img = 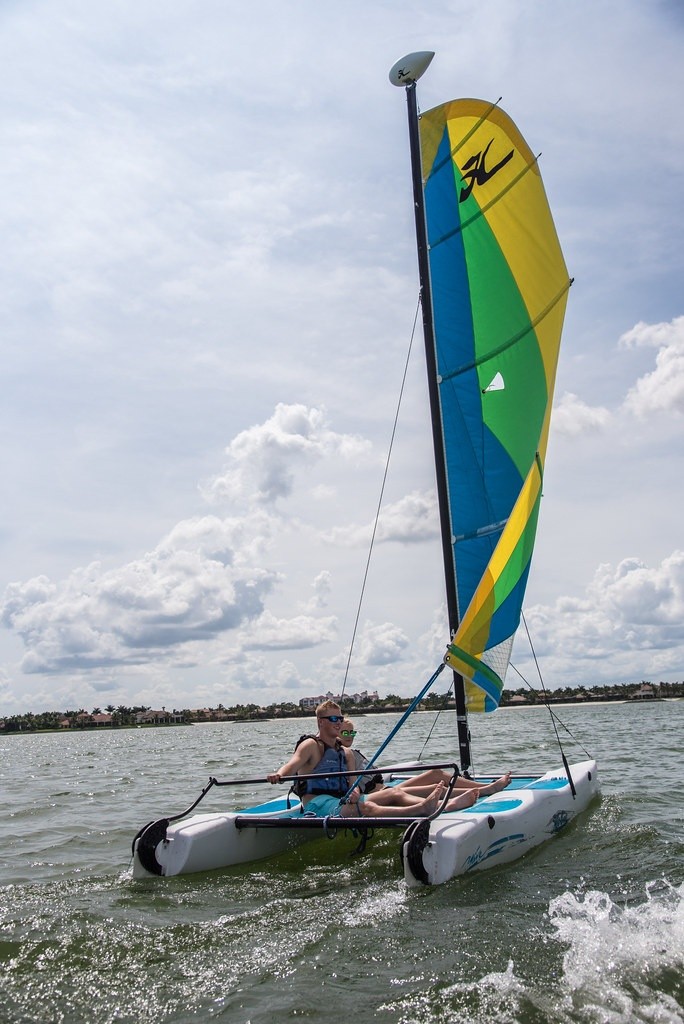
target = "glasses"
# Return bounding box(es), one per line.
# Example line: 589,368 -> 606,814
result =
320,716 -> 344,722
340,730 -> 357,737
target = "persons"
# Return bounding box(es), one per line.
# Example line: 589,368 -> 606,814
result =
335,717 -> 514,800
266,700 -> 479,819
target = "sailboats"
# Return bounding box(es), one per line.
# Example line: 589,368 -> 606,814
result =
128,46 -> 601,898
